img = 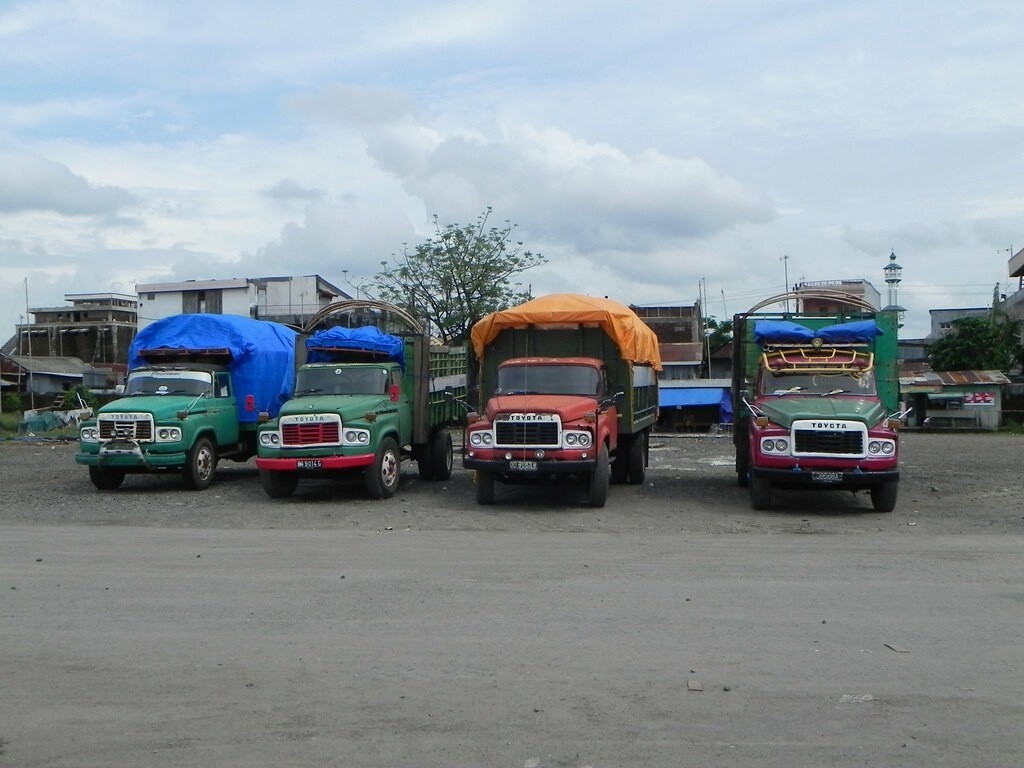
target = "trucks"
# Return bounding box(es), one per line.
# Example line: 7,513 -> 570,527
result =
247,320 -> 467,499
733,313 -> 907,510
75,315 -> 298,489
446,297 -> 658,506
